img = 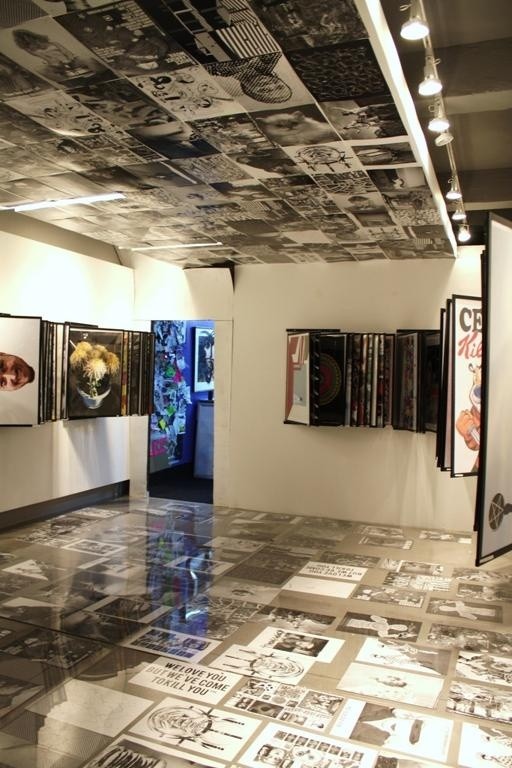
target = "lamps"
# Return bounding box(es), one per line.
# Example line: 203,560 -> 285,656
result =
398,1 -> 471,244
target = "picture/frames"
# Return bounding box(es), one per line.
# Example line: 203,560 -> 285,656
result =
0,312 -> 157,429
285,293 -> 482,479
191,325 -> 214,394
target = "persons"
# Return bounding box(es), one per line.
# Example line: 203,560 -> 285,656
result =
0,352 -> 35,391
62,609 -> 136,644
204,47 -> 292,106
12,29 -> 79,69
345,196 -> 387,216
449,655 -> 512,768
284,637 -> 329,656
236,156 -> 296,174
366,640 -> 452,675
347,615 -> 408,639
439,600 -> 495,620
329,102 -> 402,133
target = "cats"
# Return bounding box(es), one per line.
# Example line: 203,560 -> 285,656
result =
254,110 -> 336,146
